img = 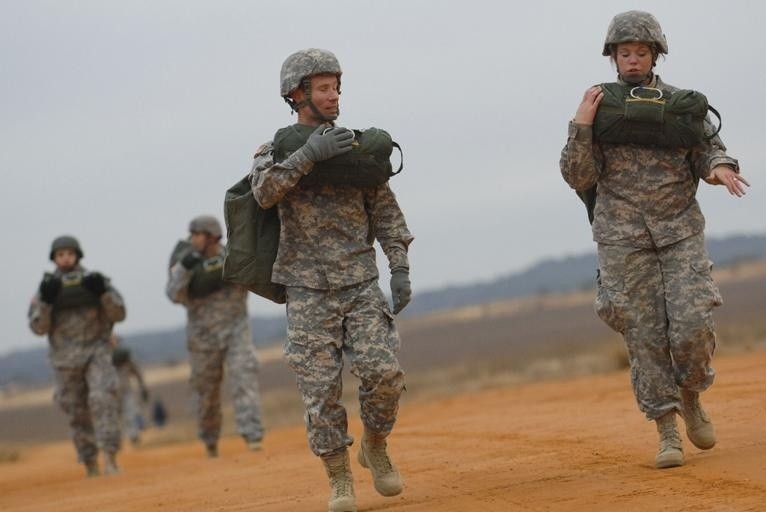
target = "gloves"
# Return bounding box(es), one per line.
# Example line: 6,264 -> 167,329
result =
40,279 -> 61,302
183,252 -> 202,270
82,275 -> 105,295
390,272 -> 411,314
302,124 -> 352,162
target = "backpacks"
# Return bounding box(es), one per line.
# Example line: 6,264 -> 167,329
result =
223,173 -> 286,304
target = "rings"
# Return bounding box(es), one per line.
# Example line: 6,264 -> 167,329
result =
731,177 -> 737,181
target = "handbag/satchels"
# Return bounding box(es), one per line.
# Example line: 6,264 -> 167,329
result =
55,281 -> 92,306
592,83 -> 708,147
188,257 -> 228,297
273,124 -> 392,187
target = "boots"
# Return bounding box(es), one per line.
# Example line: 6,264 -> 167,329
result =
322,450 -> 355,512
678,389 -> 715,449
656,410 -> 684,467
358,425 -> 402,495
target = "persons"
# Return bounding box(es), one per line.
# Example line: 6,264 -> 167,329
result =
163,216 -> 267,459
109,334 -> 149,447
26,237 -> 127,478
249,47 -> 420,511
557,9 -> 750,469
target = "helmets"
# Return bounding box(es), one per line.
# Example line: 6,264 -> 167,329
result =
602,11 -> 668,56
280,49 -> 341,96
50,236 -> 82,259
190,216 -> 222,237
